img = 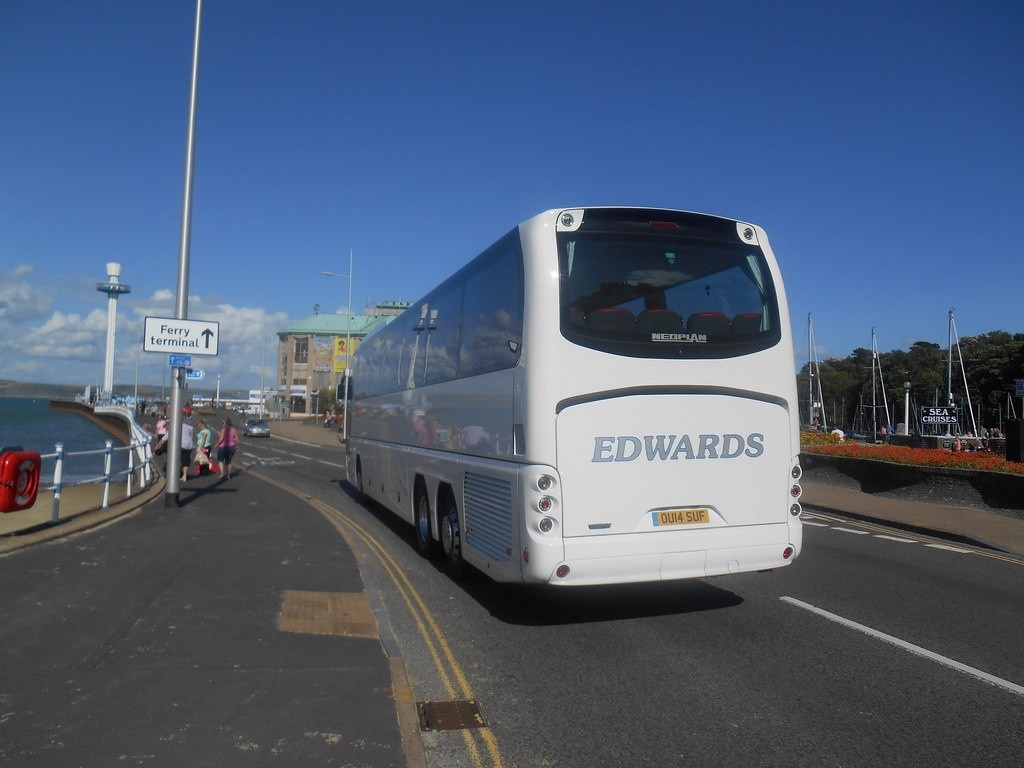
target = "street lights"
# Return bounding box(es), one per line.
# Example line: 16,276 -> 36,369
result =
321,247 -> 349,443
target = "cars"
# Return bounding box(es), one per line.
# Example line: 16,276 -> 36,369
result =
238,417 -> 271,438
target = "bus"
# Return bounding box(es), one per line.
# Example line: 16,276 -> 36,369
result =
336,204 -> 808,591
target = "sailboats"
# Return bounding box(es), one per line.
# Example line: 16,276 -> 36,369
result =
799,305 -> 1006,451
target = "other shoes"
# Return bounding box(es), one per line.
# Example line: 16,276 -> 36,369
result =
180,477 -> 186,481
226,477 -> 230,480
218,477 -> 224,479
200,472 -> 209,475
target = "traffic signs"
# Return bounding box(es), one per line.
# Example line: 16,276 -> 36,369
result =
142,316 -> 220,357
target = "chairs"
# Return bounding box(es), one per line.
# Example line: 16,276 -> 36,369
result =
732,313 -> 762,333
569,306 -> 586,328
587,308 -> 636,333
687,312 -> 730,336
636,308 -> 684,335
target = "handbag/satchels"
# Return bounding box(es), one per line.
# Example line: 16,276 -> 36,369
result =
194,448 -> 209,465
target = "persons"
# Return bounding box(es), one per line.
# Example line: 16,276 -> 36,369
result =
212,397 -> 215,407
140,400 -> 146,416
881,424 -> 892,441
945,425 -> 1003,452
338,340 -> 344,352
196,420 -> 212,477
143,417 -> 193,482
151,405 -> 157,418
183,402 -> 193,419
830,422 -> 847,442
214,418 -> 240,480
90,396 -> 93,405
325,411 -> 332,427
809,417 -> 823,434
